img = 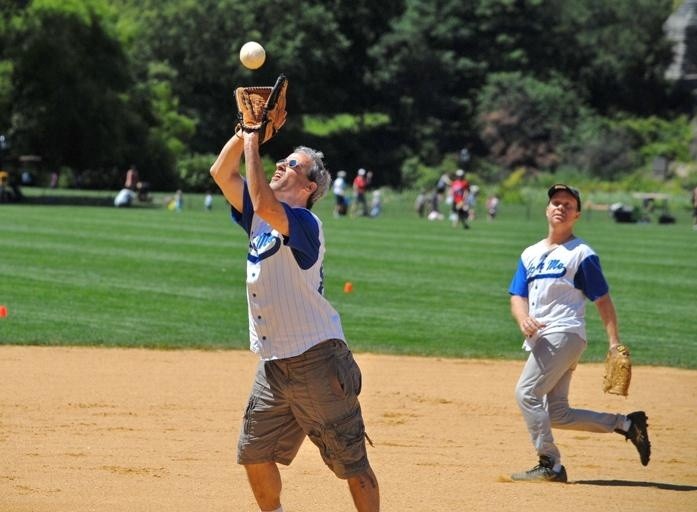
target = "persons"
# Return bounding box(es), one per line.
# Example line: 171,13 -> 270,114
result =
506,183 -> 650,481
331,170 -> 348,218
412,168 -> 503,230
351,168 -> 372,216
369,185 -> 381,216
114,185 -> 138,207
174,182 -> 184,212
124,165 -> 139,192
203,190 -> 212,211
689,186 -> 696,232
211,75 -> 382,512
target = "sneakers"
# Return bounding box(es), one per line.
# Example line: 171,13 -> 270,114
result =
511,454 -> 568,482
615,411 -> 651,466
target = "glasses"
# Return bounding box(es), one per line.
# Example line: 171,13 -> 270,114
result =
278,159 -> 302,171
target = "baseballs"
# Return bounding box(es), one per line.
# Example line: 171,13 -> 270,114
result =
239,42 -> 265,70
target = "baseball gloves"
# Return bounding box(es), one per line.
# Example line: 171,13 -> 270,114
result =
232,73 -> 288,145
602,345 -> 630,395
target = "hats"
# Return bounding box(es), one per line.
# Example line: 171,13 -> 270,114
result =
548,183 -> 581,210
